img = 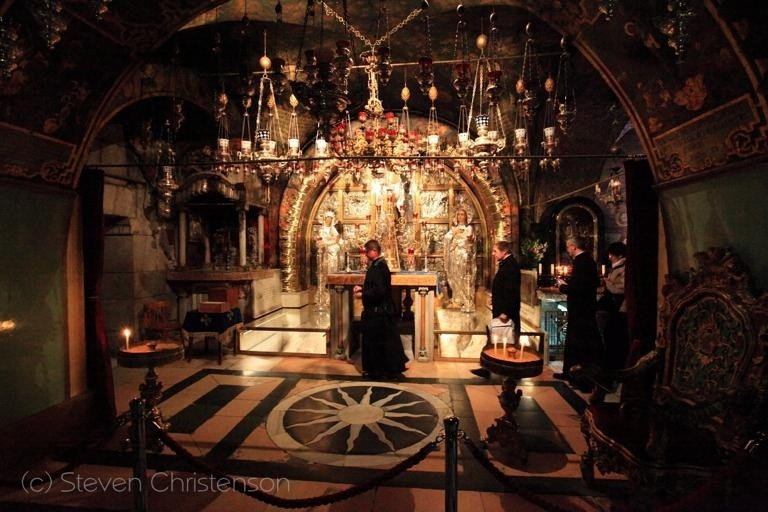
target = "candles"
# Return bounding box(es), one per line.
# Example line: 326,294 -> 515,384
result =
538,263 -> 555,275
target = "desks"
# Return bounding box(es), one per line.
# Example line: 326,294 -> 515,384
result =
186,306 -> 240,365
325,270 -> 439,363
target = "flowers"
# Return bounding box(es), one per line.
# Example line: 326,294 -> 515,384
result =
526,238 -> 550,263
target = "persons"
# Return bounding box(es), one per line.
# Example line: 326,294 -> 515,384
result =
314,211 -> 340,307
596,240 -> 624,313
553,236 -> 599,380
444,209 -> 477,312
361,241 -> 404,382
471,241 -> 520,378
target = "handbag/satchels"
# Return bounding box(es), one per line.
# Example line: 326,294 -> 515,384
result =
487,318 -> 516,344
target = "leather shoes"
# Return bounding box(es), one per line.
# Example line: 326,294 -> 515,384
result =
553,372 -> 569,380
471,368 -> 490,379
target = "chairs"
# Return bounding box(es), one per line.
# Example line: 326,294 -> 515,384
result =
579,242 -> 768,512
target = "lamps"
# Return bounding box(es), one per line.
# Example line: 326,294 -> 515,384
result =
209,0 -> 581,184
